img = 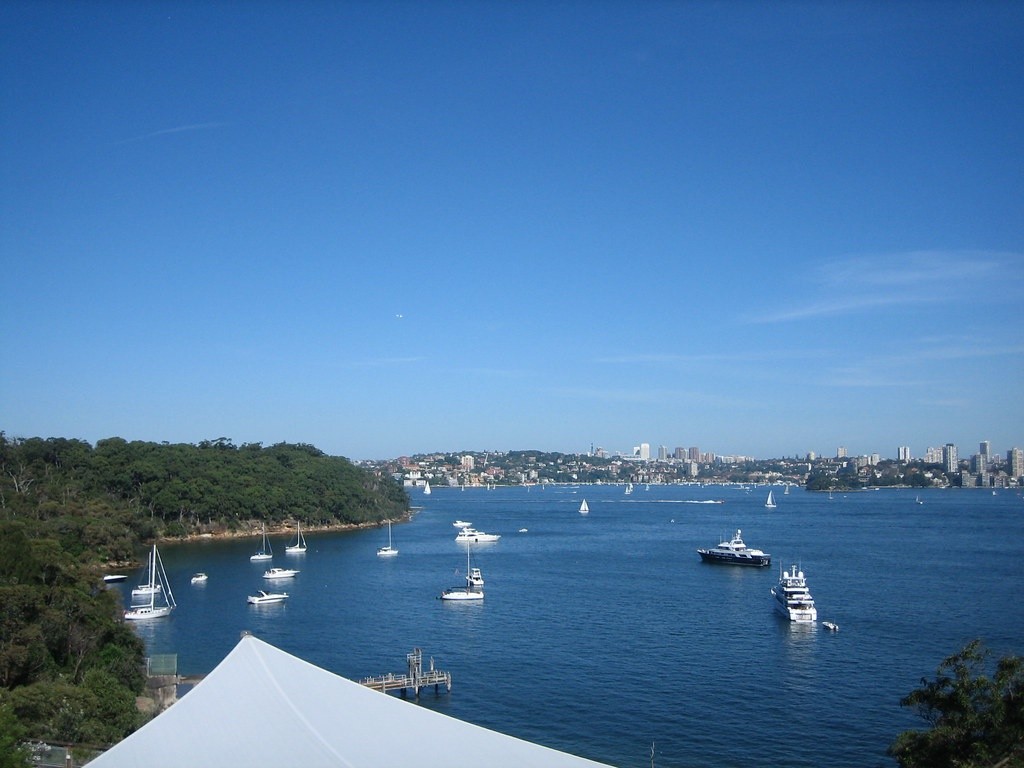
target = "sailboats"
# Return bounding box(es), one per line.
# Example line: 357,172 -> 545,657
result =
765,490 -> 777,508
125,544 -> 177,619
376,519 -> 399,555
441,539 -> 484,600
132,552 -> 161,595
286,521 -> 307,552
423,481 -> 432,494
578,499 -> 589,514
784,485 -> 789,494
249,523 -> 273,560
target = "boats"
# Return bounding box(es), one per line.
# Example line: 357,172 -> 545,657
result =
697,529 -> 772,568
822,622 -> 839,630
262,568 -> 300,579
466,568 -> 484,588
247,591 -> 290,604
453,520 -> 473,529
771,556 -> 817,624
191,573 -> 208,582
455,527 -> 501,542
104,575 -> 128,584
